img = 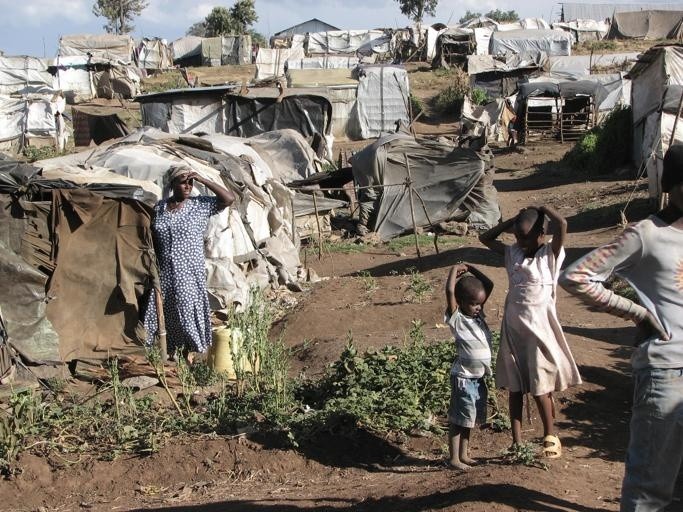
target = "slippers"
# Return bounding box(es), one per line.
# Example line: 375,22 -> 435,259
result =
541,434 -> 562,459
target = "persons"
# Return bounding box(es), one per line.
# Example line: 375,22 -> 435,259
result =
558,144 -> 683,512
444,262 -> 494,470
478,205 -> 583,462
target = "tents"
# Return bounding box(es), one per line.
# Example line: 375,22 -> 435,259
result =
1,1 -> 683,365
